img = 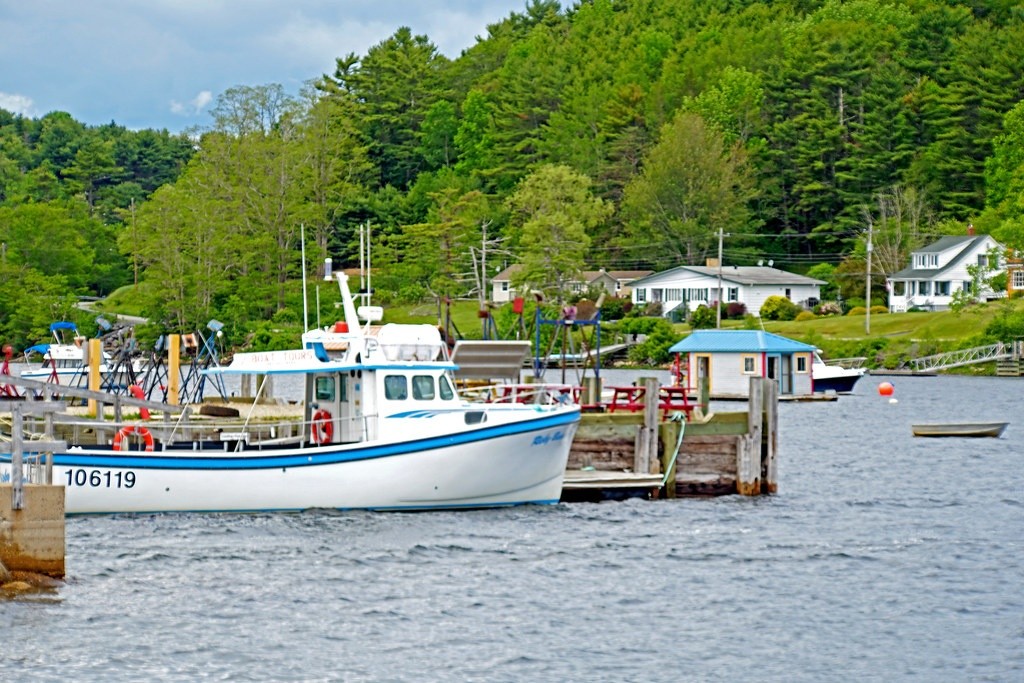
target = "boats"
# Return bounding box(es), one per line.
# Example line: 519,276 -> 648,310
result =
0,257 -> 584,515
21,321 -> 155,390
812,345 -> 867,393
911,421 -> 1011,439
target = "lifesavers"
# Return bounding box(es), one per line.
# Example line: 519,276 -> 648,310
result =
111,426 -> 153,453
311,409 -> 333,444
